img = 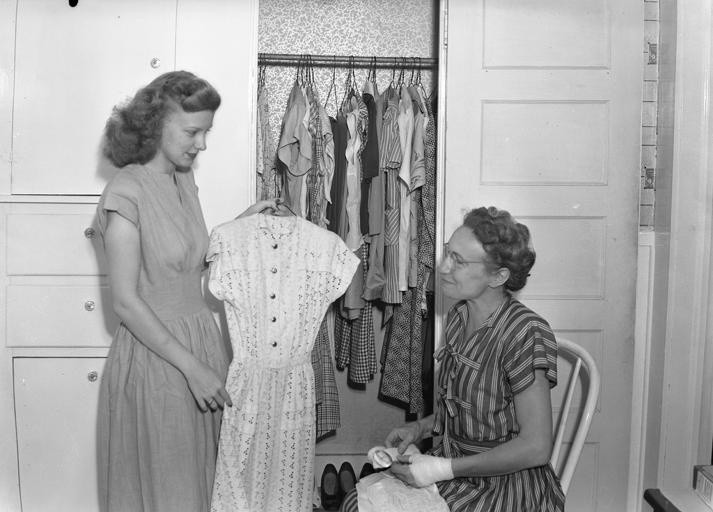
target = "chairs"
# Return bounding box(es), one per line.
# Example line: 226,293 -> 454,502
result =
537,335 -> 601,497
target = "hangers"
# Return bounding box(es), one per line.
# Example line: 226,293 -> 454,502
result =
256,185 -> 296,220
256,54 -> 438,146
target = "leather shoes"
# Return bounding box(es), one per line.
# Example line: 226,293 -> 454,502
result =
321,462 -> 357,511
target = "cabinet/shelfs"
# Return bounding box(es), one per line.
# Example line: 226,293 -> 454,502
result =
439,4 -> 646,512
6,2 -> 248,511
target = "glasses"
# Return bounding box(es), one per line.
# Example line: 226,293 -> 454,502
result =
442,242 -> 487,269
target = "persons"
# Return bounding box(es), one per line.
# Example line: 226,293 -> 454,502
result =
330,201 -> 569,511
91,69 -> 234,512
